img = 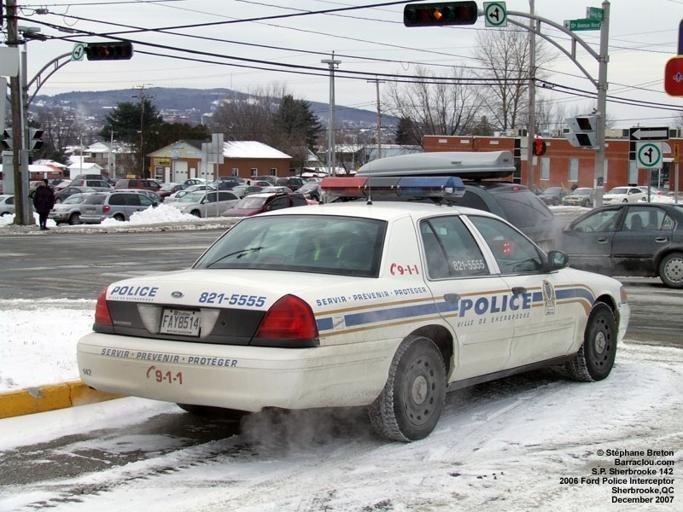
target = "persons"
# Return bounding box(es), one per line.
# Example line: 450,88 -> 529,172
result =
33,179 -> 54,230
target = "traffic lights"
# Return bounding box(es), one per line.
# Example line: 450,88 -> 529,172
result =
403,1 -> 477,28
29,129 -> 44,152
574,115 -> 598,148
85,42 -> 132,61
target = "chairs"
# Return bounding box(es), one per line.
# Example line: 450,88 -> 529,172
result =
628,214 -> 643,229
271,230 -> 471,276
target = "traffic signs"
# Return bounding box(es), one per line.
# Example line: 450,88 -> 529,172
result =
564,6 -> 605,31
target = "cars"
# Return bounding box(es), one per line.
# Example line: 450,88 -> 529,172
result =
600,185 -> 656,207
557,201 -> 682,288
